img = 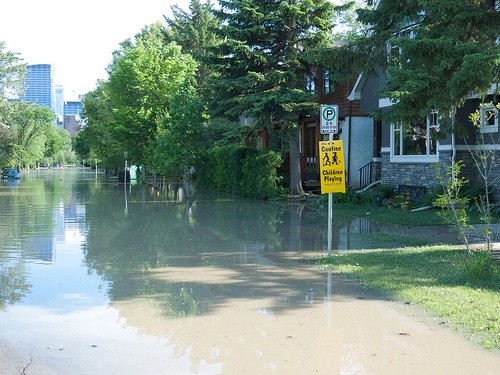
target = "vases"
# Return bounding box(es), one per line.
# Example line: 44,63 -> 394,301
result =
389,206 -> 393,209
401,202 -> 410,211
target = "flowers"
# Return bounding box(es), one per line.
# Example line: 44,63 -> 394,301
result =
396,192 -> 412,202
388,198 -> 392,206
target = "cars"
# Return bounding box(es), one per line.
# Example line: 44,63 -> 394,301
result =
0,168 -> 21,179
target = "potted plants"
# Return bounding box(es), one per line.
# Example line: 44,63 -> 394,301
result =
370,191 -> 385,206
